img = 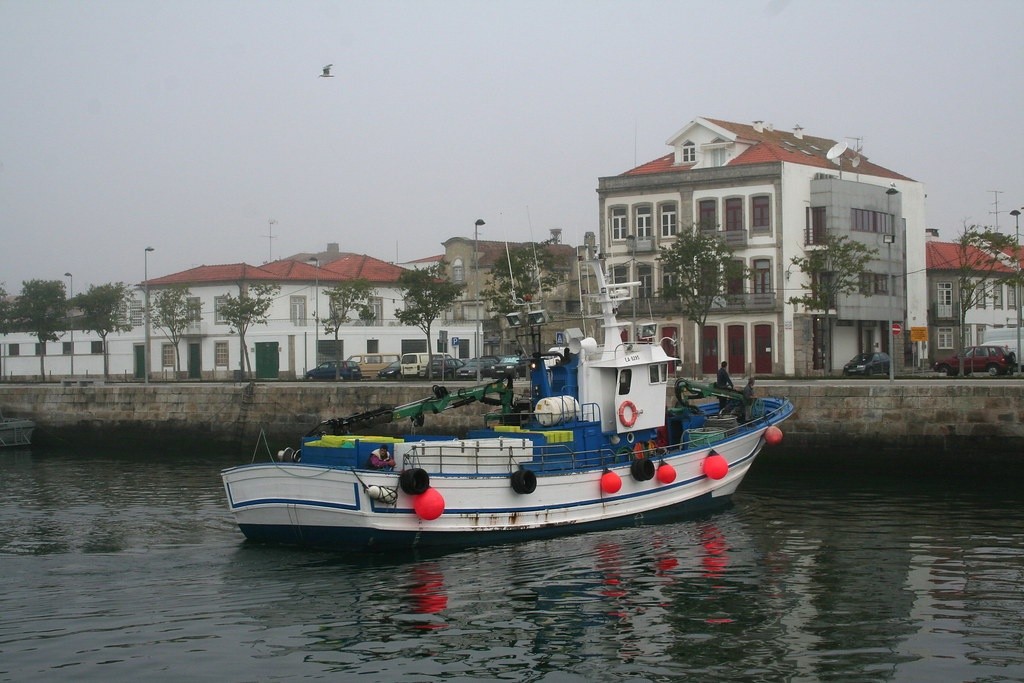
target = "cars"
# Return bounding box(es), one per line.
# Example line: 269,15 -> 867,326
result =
305,360 -> 361,381
456,355 -> 497,381
489,353 -> 533,379
427,358 -> 462,380
841,351 -> 891,376
377,361 -> 402,381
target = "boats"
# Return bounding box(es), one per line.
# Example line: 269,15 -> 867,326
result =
216,245 -> 799,549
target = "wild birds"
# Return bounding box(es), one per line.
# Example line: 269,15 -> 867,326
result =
318,64 -> 334,77
626,344 -> 633,353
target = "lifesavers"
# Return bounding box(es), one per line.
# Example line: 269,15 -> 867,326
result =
511,471 -> 525,494
631,460 -> 645,481
618,401 -> 637,427
518,469 -> 537,493
638,459 -> 655,480
399,469 -> 415,494
406,468 -> 429,494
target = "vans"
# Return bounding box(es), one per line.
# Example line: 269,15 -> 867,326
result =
967,328 -> 1024,367
345,352 -> 399,380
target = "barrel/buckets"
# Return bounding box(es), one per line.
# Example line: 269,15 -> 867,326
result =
704,414 -> 739,437
688,426 -> 724,450
534,394 -> 580,426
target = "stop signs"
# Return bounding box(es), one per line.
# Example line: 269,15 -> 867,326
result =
893,323 -> 901,335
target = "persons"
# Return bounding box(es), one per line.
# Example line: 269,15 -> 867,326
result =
743,377 -> 758,427
369,444 -> 397,471
717,361 -> 734,413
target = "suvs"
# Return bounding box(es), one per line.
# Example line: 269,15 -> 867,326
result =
934,344 -> 1016,379
400,353 -> 456,379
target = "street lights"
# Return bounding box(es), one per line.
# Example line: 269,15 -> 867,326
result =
1010,209 -> 1023,377
64,272 -> 73,377
475,218 -> 485,381
309,255 -> 318,368
885,234 -> 895,378
144,246 -> 154,386
